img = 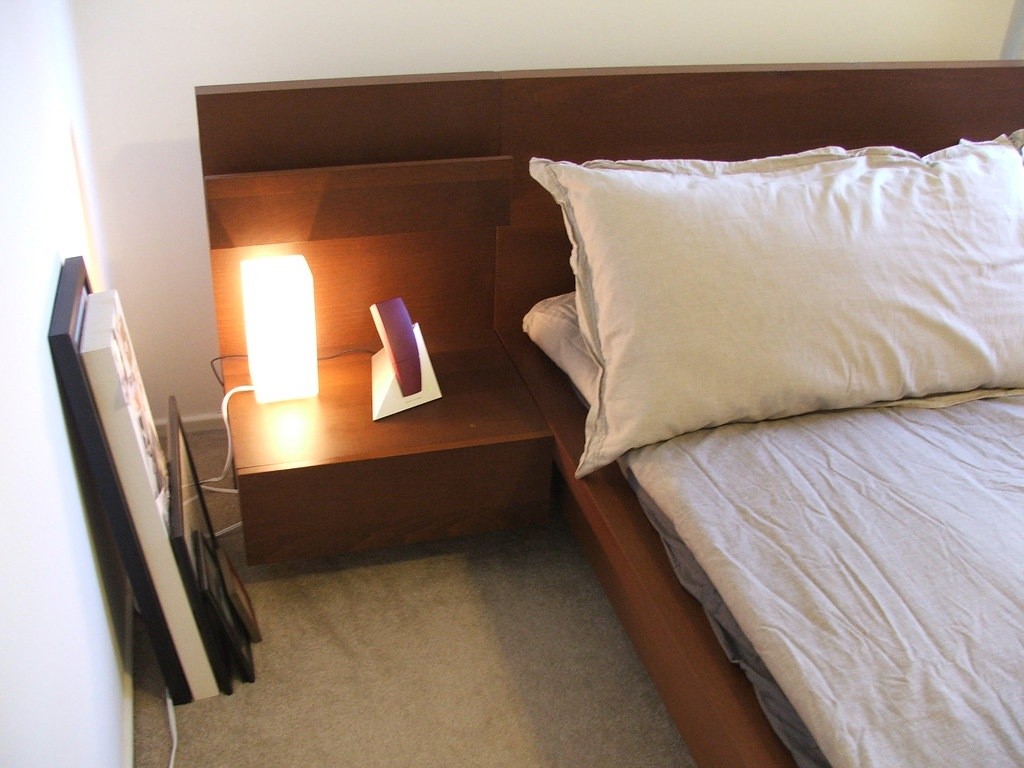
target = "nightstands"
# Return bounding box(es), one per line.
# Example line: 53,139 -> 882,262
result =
194,70 -> 557,568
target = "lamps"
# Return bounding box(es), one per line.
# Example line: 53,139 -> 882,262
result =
239,254 -> 319,407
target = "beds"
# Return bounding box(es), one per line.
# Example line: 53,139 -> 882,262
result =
497,56 -> 1024,768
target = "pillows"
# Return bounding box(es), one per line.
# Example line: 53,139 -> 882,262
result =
526,131 -> 1024,480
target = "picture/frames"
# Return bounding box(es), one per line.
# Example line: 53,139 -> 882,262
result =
47,254 -> 263,702
